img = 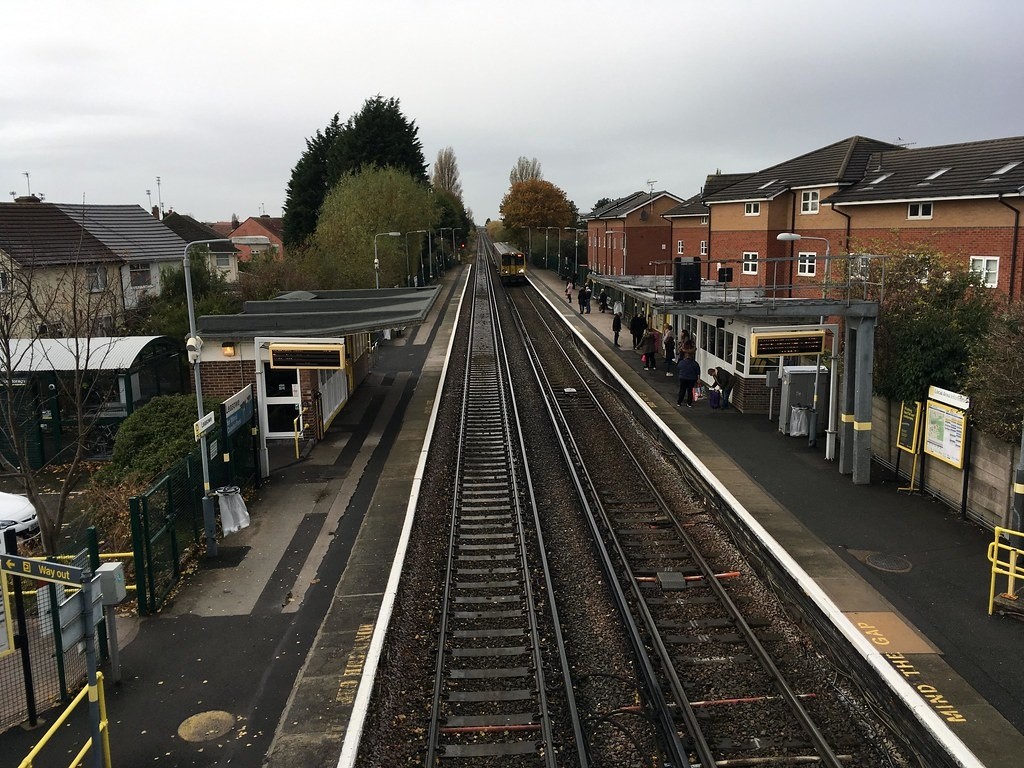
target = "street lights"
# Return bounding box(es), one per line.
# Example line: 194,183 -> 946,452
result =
405,230 -> 427,287
453,227 -> 462,253
520,225 -> 532,263
605,230 -> 627,276
777,233 -> 831,447
537,226 -> 548,269
183,236 -> 271,558
440,227 -> 453,268
564,227 -> 578,274
374,232 -> 401,290
577,228 -> 595,272
548,226 -> 561,275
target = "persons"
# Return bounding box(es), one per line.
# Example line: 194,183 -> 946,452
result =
662,323 -> 675,376
708,366 -> 736,410
612,312 -> 622,347
578,282 -> 592,314
676,353 -> 700,407
599,288 -> 607,313
635,328 -> 656,371
640,313 -> 647,339
630,312 -> 644,350
677,329 -> 690,363
566,277 -> 573,303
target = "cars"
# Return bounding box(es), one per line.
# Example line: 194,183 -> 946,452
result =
0,491 -> 41,549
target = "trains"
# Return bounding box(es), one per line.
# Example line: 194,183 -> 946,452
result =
493,241 -> 526,280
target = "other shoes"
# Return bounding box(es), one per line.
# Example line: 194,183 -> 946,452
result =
643,367 -> 649,370
601,310 -> 605,313
580,312 -> 583,314
721,407 -> 725,409
653,368 -> 656,370
614,344 -> 620,347
585,312 -> 588,314
666,372 -> 673,376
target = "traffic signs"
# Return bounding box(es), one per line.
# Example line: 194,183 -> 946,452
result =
0,554 -> 83,588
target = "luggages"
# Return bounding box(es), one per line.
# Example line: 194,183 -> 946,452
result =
709,390 -> 720,409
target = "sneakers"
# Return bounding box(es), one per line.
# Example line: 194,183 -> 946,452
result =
686,404 -> 691,408
676,402 -> 681,407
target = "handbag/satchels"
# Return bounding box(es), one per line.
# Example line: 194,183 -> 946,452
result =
692,386 -> 707,402
641,354 -> 646,362
565,289 -> 568,293
